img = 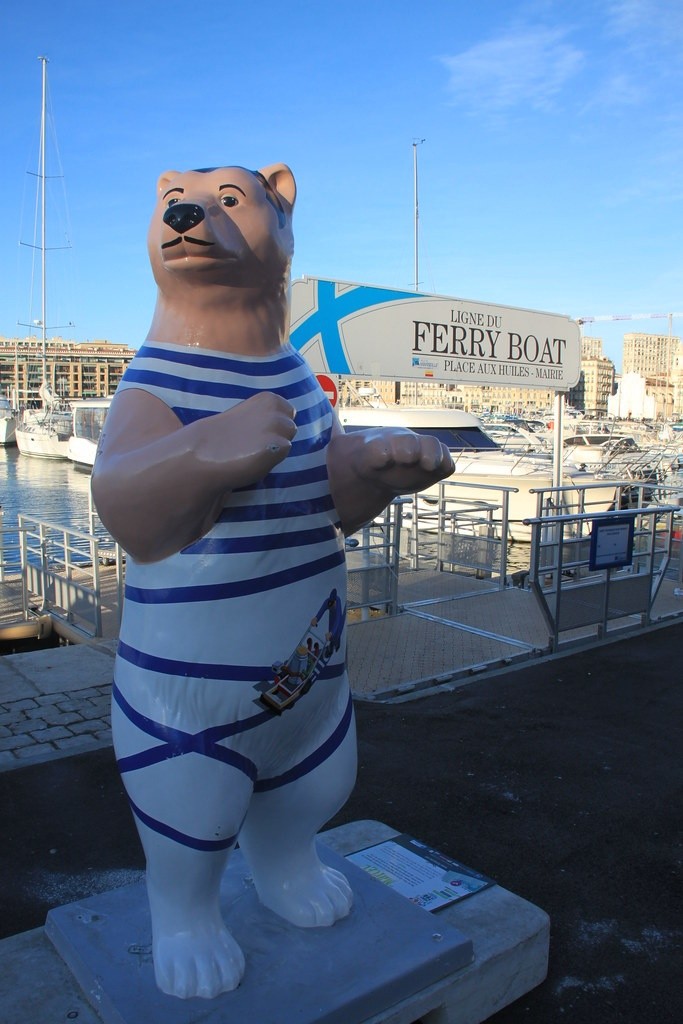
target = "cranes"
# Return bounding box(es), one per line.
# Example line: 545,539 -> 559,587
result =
574,312 -> 682,334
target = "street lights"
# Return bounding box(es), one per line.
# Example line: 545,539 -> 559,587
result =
412,138 -> 426,293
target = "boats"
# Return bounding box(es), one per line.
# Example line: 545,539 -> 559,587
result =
3,388 -> 683,555
